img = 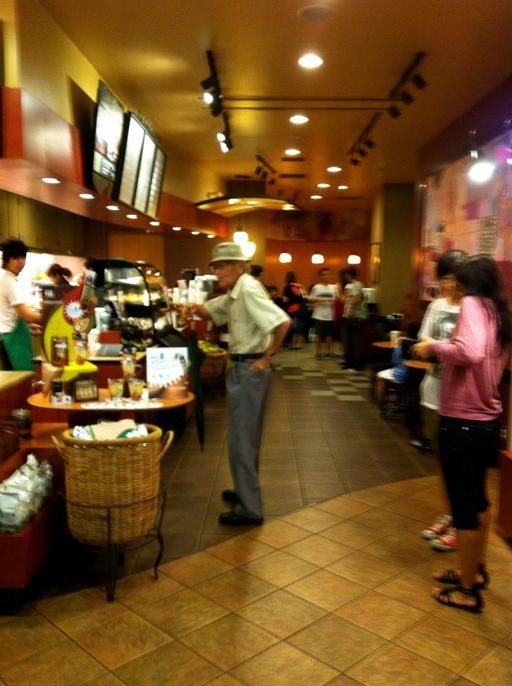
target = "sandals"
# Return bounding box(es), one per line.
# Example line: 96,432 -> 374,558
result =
437,562 -> 491,589
432,583 -> 485,614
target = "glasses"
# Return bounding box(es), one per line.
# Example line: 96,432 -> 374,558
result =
209,261 -> 224,273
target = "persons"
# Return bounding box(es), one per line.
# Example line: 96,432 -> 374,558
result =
395,292 -> 426,329
177,240 -> 294,529
46,262 -> 74,287
250,261 -> 372,374
415,247 -> 473,550
390,321 -> 422,407
410,254 -> 506,615
0,235 -> 43,370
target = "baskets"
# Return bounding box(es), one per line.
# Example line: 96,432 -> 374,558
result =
51,423 -> 175,549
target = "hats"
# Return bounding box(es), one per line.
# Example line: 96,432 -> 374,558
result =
208,242 -> 252,265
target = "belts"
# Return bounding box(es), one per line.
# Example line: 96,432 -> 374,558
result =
231,353 -> 263,362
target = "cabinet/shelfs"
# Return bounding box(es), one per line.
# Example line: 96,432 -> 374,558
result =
92,253 -> 175,340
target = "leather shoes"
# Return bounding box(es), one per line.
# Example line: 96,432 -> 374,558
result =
222,490 -> 240,507
218,511 -> 263,527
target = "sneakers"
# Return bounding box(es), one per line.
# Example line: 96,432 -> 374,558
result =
429,527 -> 457,551
420,514 -> 453,538
288,347 -> 348,365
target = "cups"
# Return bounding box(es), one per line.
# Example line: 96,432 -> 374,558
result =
172,279 -> 206,323
106,349 -> 145,402
94,306 -> 112,332
390,330 -> 401,347
